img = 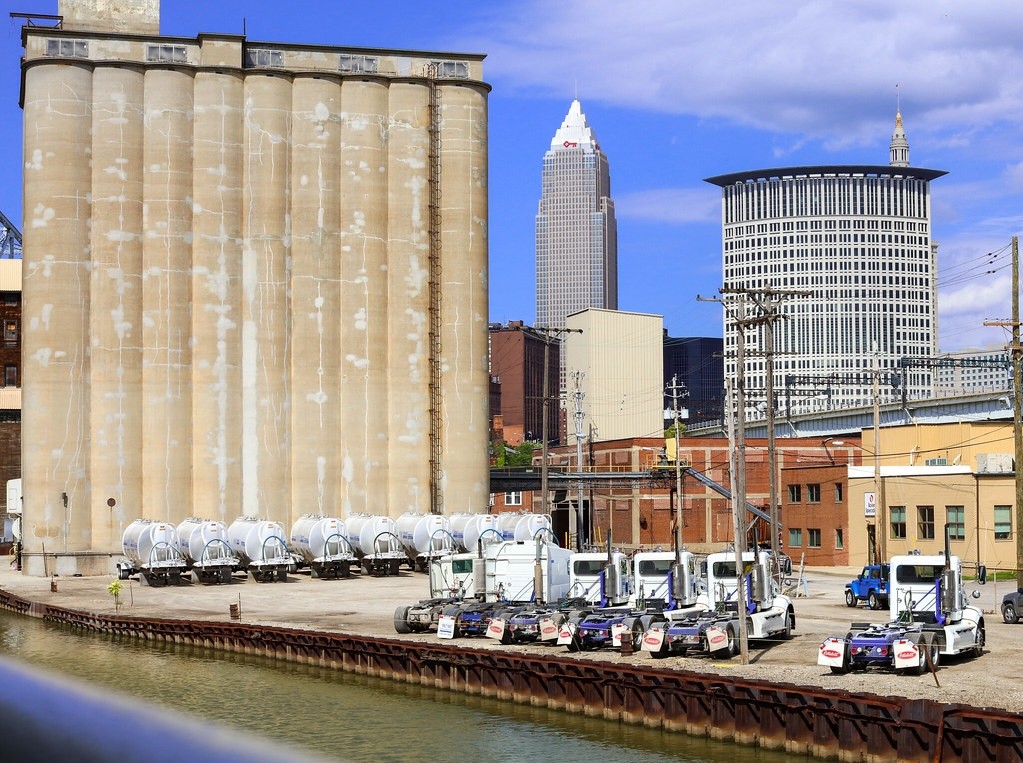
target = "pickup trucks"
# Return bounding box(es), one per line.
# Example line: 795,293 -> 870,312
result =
843,563 -> 933,611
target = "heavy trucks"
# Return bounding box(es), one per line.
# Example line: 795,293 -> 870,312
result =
817,522 -> 986,676
392,533 -> 700,655
640,513 -> 797,662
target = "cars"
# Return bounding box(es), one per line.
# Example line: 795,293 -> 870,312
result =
1001,587 -> 1023,623
